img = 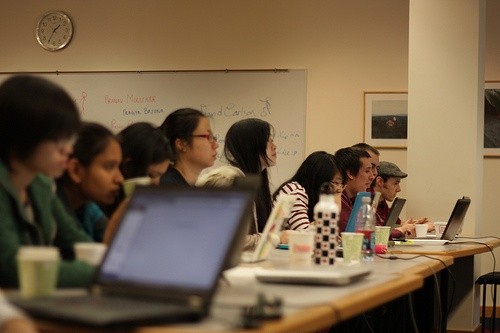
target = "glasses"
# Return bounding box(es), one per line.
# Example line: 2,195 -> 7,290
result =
191,133 -> 218,143
330,180 -> 346,190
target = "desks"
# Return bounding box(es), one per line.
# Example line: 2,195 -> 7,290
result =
37,234 -> 500,333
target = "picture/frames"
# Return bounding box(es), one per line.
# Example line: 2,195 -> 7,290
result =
364,93 -> 408,150
484,81 -> 499,158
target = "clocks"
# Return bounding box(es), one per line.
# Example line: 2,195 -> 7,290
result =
36,10 -> 74,52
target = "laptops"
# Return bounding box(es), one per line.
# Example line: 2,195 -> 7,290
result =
8,183 -> 247,326
370,191 -> 382,220
385,198 -> 406,239
404,197 -> 468,240
345,191 -> 370,231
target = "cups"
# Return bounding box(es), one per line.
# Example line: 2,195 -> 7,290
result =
415,224 -> 427,239
124,177 -> 150,192
342,232 -> 364,263
14,246 -> 61,297
435,222 -> 447,238
74,242 -> 108,266
286,229 -> 314,256
375,227 -> 392,244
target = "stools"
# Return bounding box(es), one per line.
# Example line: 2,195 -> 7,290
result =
476,272 -> 500,333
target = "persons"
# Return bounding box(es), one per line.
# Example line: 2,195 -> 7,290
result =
108,121 -> 170,218
57,122 -> 122,242
0,76 -> 129,291
333,143 -> 436,241
158,108 -> 219,188
195,117 -> 295,250
271,150 -> 347,233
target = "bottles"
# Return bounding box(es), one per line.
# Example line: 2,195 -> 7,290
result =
354,195 -> 375,254
314,182 -> 340,265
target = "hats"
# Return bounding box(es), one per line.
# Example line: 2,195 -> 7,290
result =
377,160 -> 408,178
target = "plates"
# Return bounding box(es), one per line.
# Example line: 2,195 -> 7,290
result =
409,238 -> 448,246
